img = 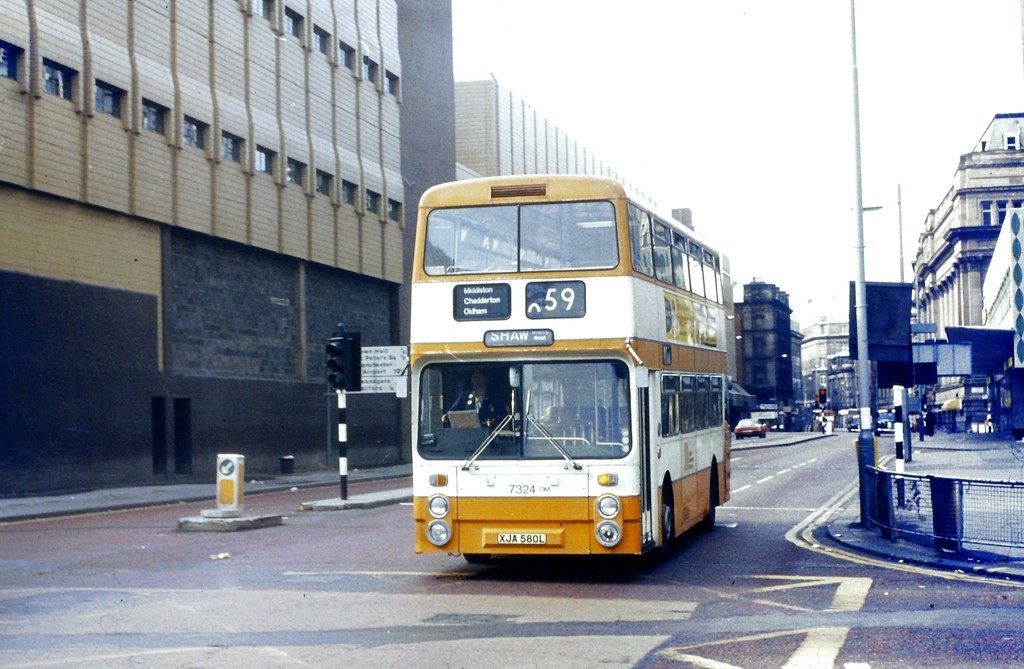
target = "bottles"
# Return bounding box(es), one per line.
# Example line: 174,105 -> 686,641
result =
487,418 -> 494,428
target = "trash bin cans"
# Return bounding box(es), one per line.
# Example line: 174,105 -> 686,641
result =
278,457 -> 295,474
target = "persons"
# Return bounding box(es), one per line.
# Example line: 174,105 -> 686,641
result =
913,419 -> 919,432
440,369 -> 506,429
925,413 -> 935,436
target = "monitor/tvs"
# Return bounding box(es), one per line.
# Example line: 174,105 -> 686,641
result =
448,410 -> 481,428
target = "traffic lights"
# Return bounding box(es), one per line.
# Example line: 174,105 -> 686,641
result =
819,389 -> 827,404
325,335 -> 350,391
815,395 -> 821,408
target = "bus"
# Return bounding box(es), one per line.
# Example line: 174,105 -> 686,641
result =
400,172 -> 733,572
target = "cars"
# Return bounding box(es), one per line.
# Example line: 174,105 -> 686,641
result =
846,418 -> 860,433
876,409 -> 896,436
733,418 -> 766,438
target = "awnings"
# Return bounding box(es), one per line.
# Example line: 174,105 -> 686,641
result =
945,326 -> 1015,378
